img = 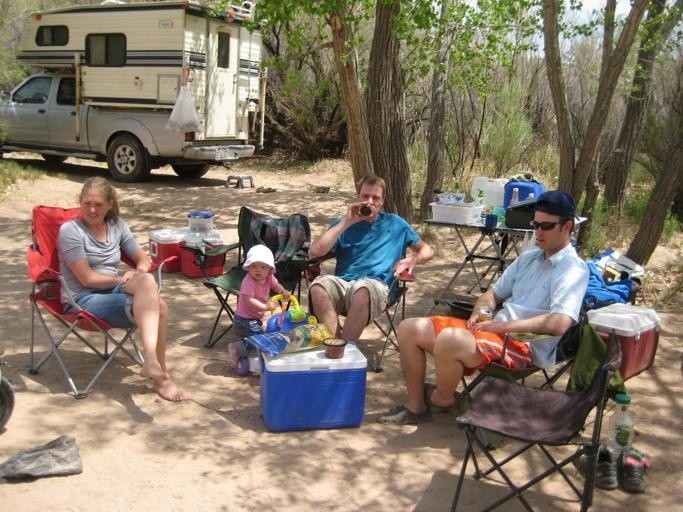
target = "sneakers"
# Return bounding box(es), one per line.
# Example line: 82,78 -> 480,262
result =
618,448 -> 648,493
575,442 -> 619,490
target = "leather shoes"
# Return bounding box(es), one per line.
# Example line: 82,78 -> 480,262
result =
424,382 -> 466,412
379,406 -> 433,425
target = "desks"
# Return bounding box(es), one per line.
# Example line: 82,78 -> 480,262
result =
422,216 -> 589,316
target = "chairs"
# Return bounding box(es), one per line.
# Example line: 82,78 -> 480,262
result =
449,310 -> 624,512
306,250 -> 416,372
28,206 -> 144,397
203,207 -> 311,347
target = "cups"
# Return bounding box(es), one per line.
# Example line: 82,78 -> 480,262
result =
437,192 -> 463,205
324,339 -> 347,358
481,214 -> 497,229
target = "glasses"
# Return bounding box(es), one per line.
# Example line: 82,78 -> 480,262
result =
530,221 -> 563,232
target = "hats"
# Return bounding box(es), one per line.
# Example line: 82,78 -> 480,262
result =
536,191 -> 582,222
244,245 -> 278,276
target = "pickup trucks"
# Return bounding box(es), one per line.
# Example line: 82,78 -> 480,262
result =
4,69 -> 271,183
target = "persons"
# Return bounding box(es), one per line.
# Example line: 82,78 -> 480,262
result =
375,190 -> 589,426
307,170 -> 438,347
55,176 -> 190,404
227,243 -> 296,377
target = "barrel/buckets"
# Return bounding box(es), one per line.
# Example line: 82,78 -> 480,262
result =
471,176 -> 509,210
503,179 -> 544,212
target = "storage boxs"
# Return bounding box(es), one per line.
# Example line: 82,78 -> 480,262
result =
587,302 -> 661,379
259,342 -> 368,431
149,212 -> 227,278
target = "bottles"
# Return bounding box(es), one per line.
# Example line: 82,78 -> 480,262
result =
527,193 -> 535,200
492,207 -> 504,221
187,211 -> 213,240
510,188 -> 520,205
360,205 -> 372,216
606,395 -> 632,455
